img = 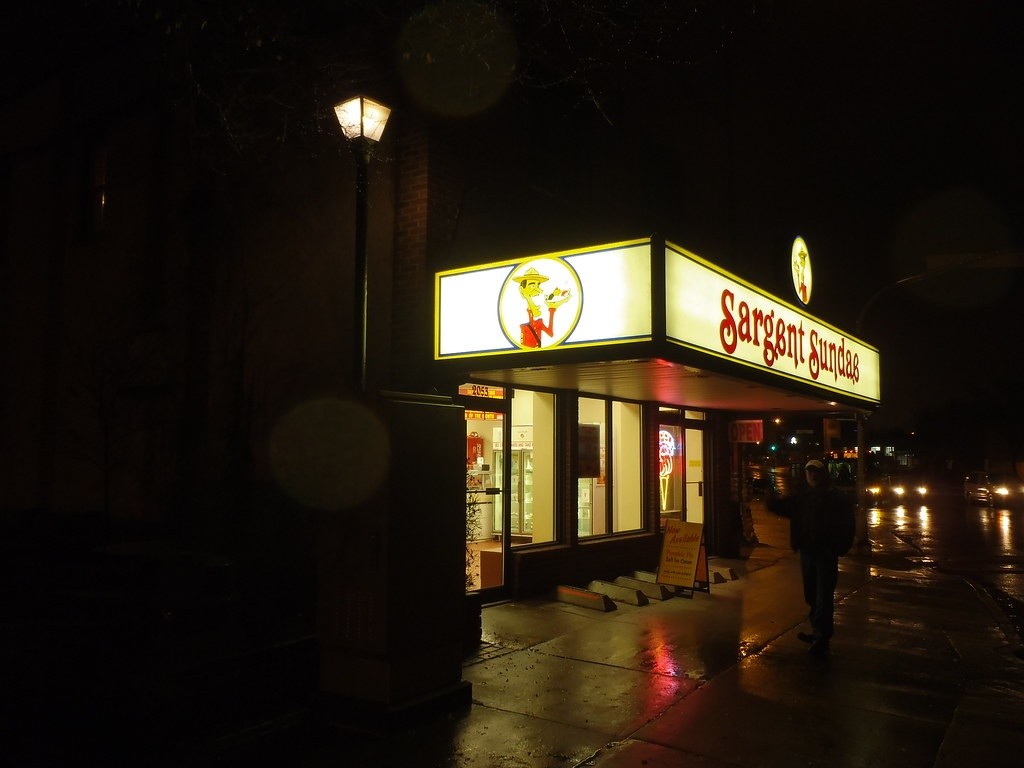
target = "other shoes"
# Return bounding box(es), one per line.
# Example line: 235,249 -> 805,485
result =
797,632 -> 815,644
808,639 -> 828,657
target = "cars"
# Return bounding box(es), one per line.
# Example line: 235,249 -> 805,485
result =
965,471 -> 1024,506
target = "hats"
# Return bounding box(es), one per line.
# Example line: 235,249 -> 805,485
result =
805,459 -> 826,472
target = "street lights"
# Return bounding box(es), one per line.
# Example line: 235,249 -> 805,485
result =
333,78 -> 393,703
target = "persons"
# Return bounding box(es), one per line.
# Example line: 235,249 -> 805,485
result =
788,460 -> 858,655
